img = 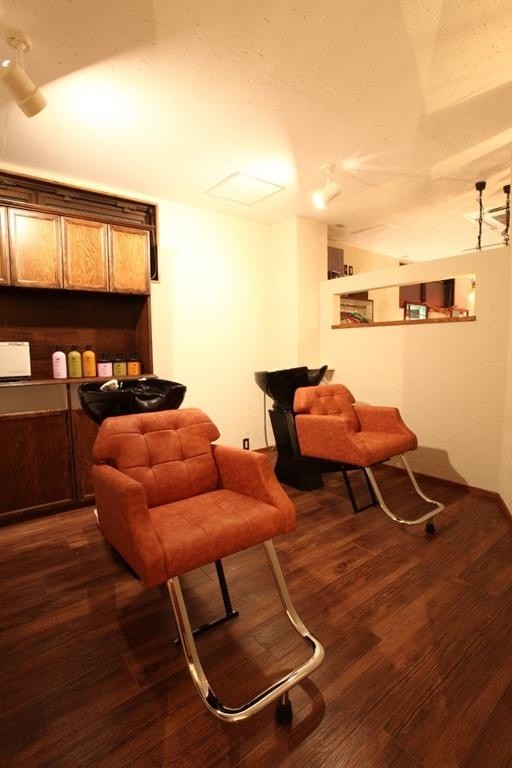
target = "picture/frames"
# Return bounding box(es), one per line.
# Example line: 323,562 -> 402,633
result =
338,295 -> 374,324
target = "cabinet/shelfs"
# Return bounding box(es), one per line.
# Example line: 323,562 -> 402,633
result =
69,369 -> 160,510
8,199 -> 64,291
326,243 -> 346,282
64,206 -> 112,295
0,378 -> 76,521
0,196 -> 11,287
104,216 -> 155,298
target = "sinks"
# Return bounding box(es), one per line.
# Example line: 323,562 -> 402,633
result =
255,365 -> 328,402
78,378 -> 186,424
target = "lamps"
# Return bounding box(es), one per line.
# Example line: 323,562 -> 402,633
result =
307,162 -> 343,212
3,23 -> 54,120
457,179 -> 488,309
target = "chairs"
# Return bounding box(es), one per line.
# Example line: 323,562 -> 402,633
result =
91,403 -> 327,731
288,377 -> 450,545
